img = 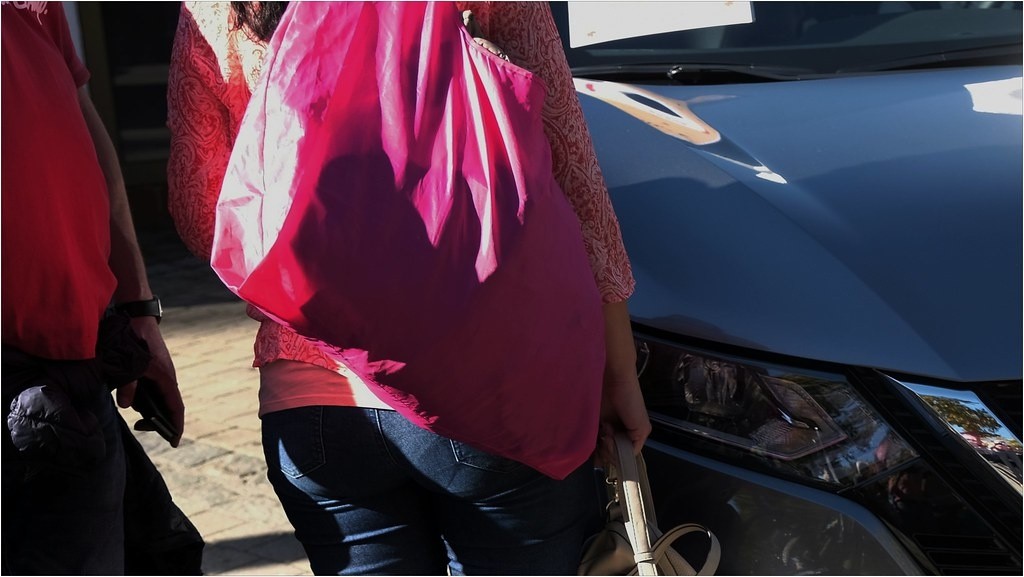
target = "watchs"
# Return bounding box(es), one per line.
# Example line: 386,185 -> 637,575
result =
111,295 -> 163,323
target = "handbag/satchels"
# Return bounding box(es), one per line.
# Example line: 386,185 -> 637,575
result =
574,431 -> 720,575
207,0 -> 602,475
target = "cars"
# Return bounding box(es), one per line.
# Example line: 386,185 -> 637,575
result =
547,3 -> 1023,575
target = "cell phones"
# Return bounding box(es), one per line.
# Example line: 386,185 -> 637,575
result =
128,380 -> 178,454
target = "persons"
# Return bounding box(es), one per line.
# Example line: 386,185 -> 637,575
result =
170,1 -> 722,577
1,1 -> 205,577
